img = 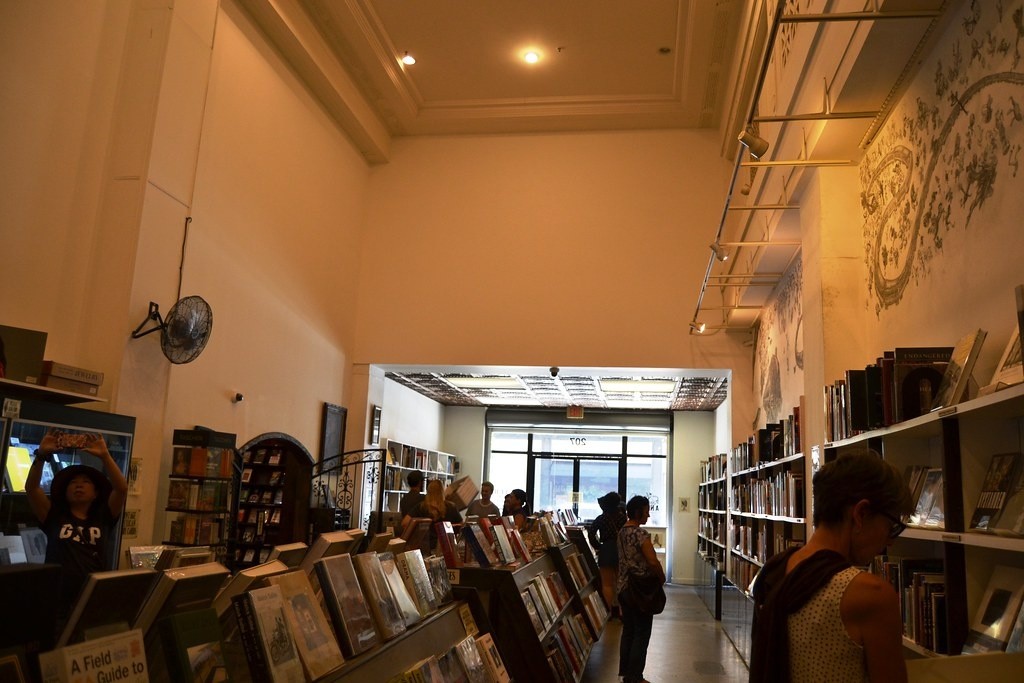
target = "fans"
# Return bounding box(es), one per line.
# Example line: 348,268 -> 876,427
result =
131,295 -> 214,365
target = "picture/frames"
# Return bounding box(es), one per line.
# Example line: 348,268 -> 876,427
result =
317,401 -> 349,476
370,404 -> 382,446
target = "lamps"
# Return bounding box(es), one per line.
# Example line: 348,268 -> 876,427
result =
688,321 -> 706,333
737,130 -> 770,159
709,241 -> 731,264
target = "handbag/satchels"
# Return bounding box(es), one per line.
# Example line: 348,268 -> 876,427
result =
617,573 -> 666,618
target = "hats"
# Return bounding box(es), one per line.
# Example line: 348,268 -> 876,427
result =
598,491 -> 621,512
50,465 -> 111,507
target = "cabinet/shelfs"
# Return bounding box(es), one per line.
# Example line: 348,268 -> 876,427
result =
310,507 -> 351,540
380,438 -> 458,513
721,574 -> 754,666
824,382 -> 1024,683
697,476 -> 728,572
693,551 -> 726,620
233,443 -> 288,570
161,428 -> 237,547
728,452 -> 805,603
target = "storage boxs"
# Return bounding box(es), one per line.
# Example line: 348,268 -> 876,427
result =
443,474 -> 480,512
40,360 -> 104,386
42,374 -> 99,396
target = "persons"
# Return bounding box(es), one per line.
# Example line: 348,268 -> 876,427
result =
502,494 -> 514,517
618,495 -> 665,683
747,449 -> 914,683
26,425 -> 128,573
588,493 -> 625,620
464,481 -> 499,520
509,489 -> 531,528
401,470 -> 428,517
401,479 -> 463,533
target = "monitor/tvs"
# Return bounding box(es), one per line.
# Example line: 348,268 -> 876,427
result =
4,440 -> 83,495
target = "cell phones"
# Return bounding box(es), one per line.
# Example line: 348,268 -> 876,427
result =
57,433 -> 87,448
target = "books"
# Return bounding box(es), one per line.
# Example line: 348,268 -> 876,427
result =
821,282 -> 1024,683
696,397 -> 806,603
0,444 -> 608,683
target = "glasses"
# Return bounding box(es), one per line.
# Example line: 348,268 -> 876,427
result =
873,507 -> 905,538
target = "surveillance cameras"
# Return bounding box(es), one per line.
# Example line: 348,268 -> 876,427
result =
231,391 -> 243,404
550,368 -> 559,377
